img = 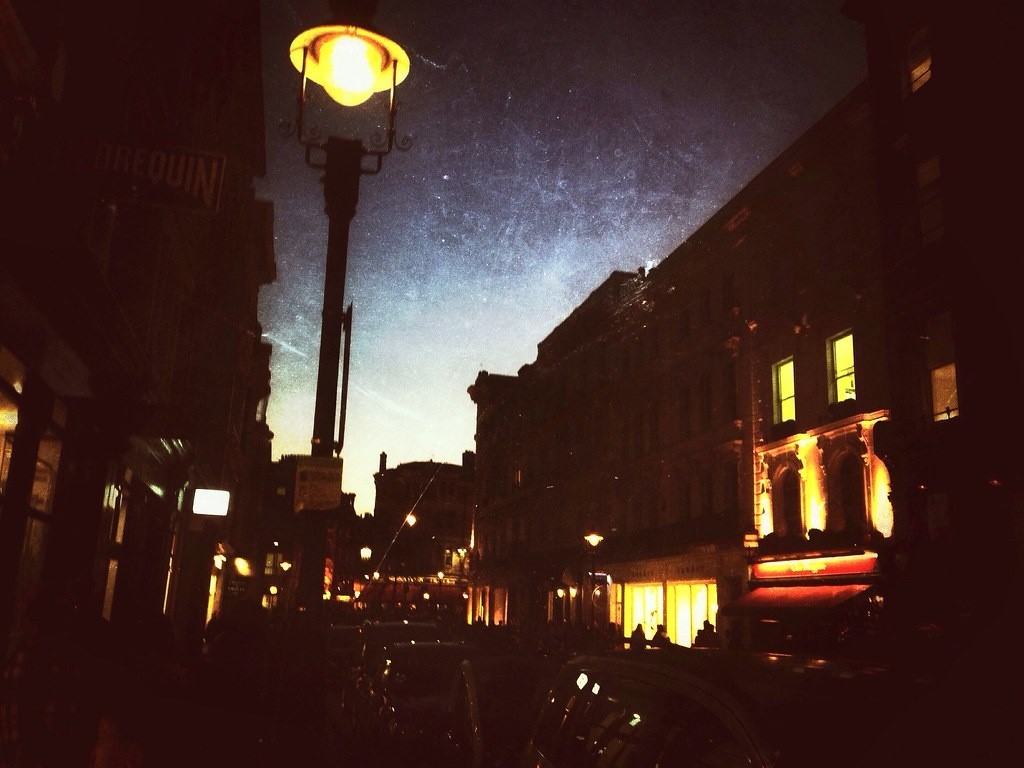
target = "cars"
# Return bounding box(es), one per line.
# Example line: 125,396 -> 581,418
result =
340,620 -> 565,768
521,648 -> 932,768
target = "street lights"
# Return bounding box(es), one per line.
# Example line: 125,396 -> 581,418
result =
291,0 -> 413,653
583,533 -> 604,656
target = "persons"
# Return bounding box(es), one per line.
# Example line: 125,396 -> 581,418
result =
543,618 -> 714,649
